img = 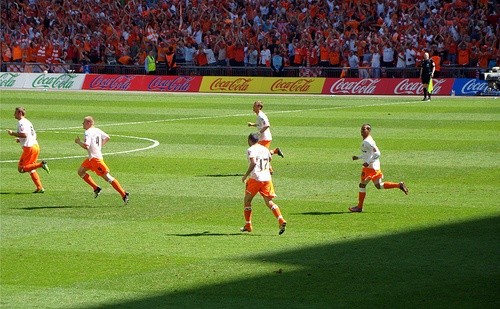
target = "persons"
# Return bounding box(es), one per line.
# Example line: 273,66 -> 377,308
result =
247,100 -> 284,175
75,116 -> 130,205
348,123 -> 408,212
419,52 -> 436,102
5,107 -> 51,193
0,0 -> 500,77
239,132 -> 287,236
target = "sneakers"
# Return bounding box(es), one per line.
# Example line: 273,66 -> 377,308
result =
278,221 -> 287,235
41,160 -> 50,173
240,226 -> 252,232
399,181 -> 409,195
122,192 -> 129,204
32,187 -> 45,193
348,206 -> 362,212
94,186 -> 101,199
276,147 -> 284,158
